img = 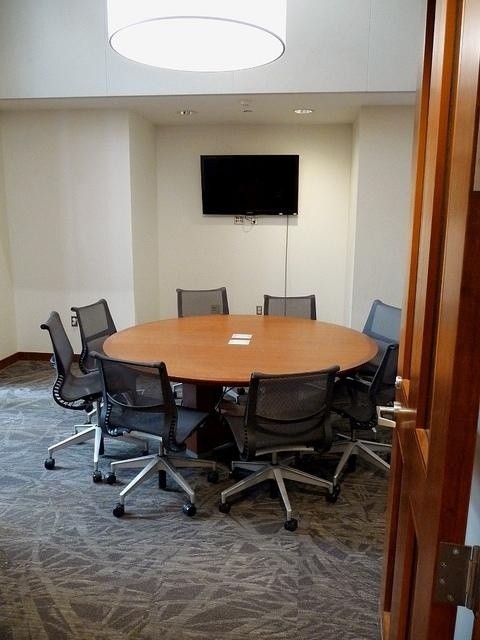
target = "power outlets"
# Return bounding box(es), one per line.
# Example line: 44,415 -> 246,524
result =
245,216 -> 258,225
234,215 -> 244,224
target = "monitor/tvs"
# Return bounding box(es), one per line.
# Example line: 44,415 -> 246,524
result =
199,154 -> 300,217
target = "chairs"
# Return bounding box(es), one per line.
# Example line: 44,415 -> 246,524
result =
321,343 -> 393,496
262,294 -> 329,404
219,365 -> 336,531
40,311 -> 149,483
73,298 -> 145,424
334,300 -> 402,407
173,287 -> 242,408
88,350 -> 221,517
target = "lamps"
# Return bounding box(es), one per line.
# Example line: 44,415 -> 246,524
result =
108,2 -> 289,73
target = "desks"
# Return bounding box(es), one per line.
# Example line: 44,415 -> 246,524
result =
102,315 -> 380,458
